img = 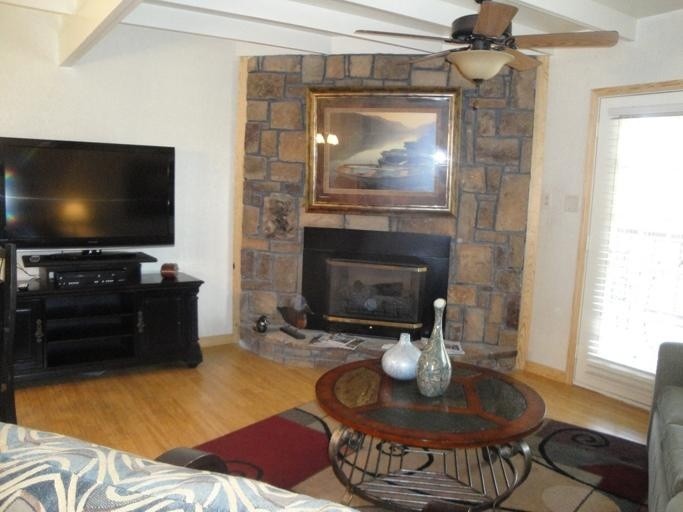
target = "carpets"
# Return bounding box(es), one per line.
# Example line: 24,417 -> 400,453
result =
190,399 -> 649,512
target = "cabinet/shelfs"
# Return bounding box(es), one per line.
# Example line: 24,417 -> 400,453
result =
12,272 -> 204,390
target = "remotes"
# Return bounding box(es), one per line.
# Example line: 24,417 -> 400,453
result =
279,325 -> 306,339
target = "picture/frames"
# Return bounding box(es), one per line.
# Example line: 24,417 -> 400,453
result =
304,85 -> 462,219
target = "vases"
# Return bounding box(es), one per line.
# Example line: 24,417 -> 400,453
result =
382,298 -> 453,397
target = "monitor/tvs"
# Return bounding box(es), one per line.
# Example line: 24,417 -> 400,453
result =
0,137 -> 176,251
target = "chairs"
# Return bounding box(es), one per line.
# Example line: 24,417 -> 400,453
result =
647,341 -> 683,512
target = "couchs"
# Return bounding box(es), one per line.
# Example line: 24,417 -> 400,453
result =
0,421 -> 360,512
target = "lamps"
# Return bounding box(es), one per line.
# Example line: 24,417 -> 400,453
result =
446,43 -> 516,93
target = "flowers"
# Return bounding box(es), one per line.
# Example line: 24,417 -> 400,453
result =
353,1 -> 620,66
